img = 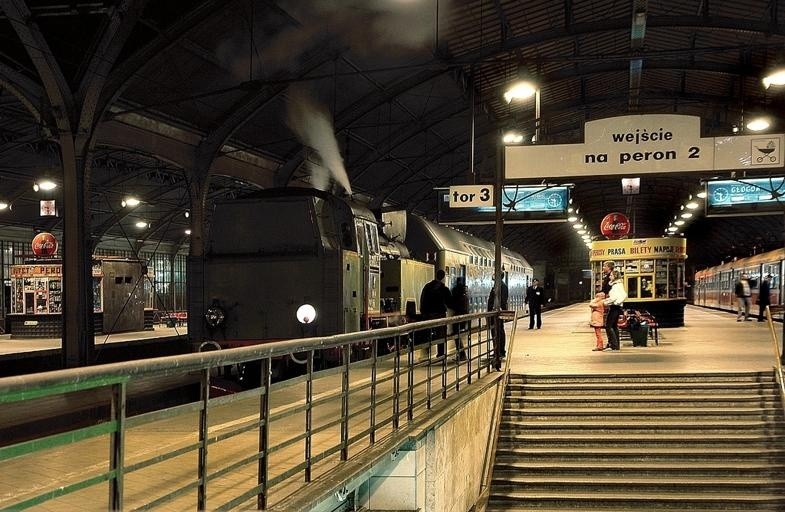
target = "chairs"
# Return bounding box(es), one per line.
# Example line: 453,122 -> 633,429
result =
160,312 -> 187,327
618,307 -> 659,347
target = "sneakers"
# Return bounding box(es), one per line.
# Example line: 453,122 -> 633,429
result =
592,347 -> 620,352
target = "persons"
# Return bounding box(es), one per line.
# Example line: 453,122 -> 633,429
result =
735,273 -> 753,321
420,269 -> 469,366
525,278 -> 544,329
488,271 -> 508,359
757,276 -> 772,322
589,261 -> 627,352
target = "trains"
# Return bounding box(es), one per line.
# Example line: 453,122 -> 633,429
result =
183,186 -> 535,388
686,246 -> 785,324
